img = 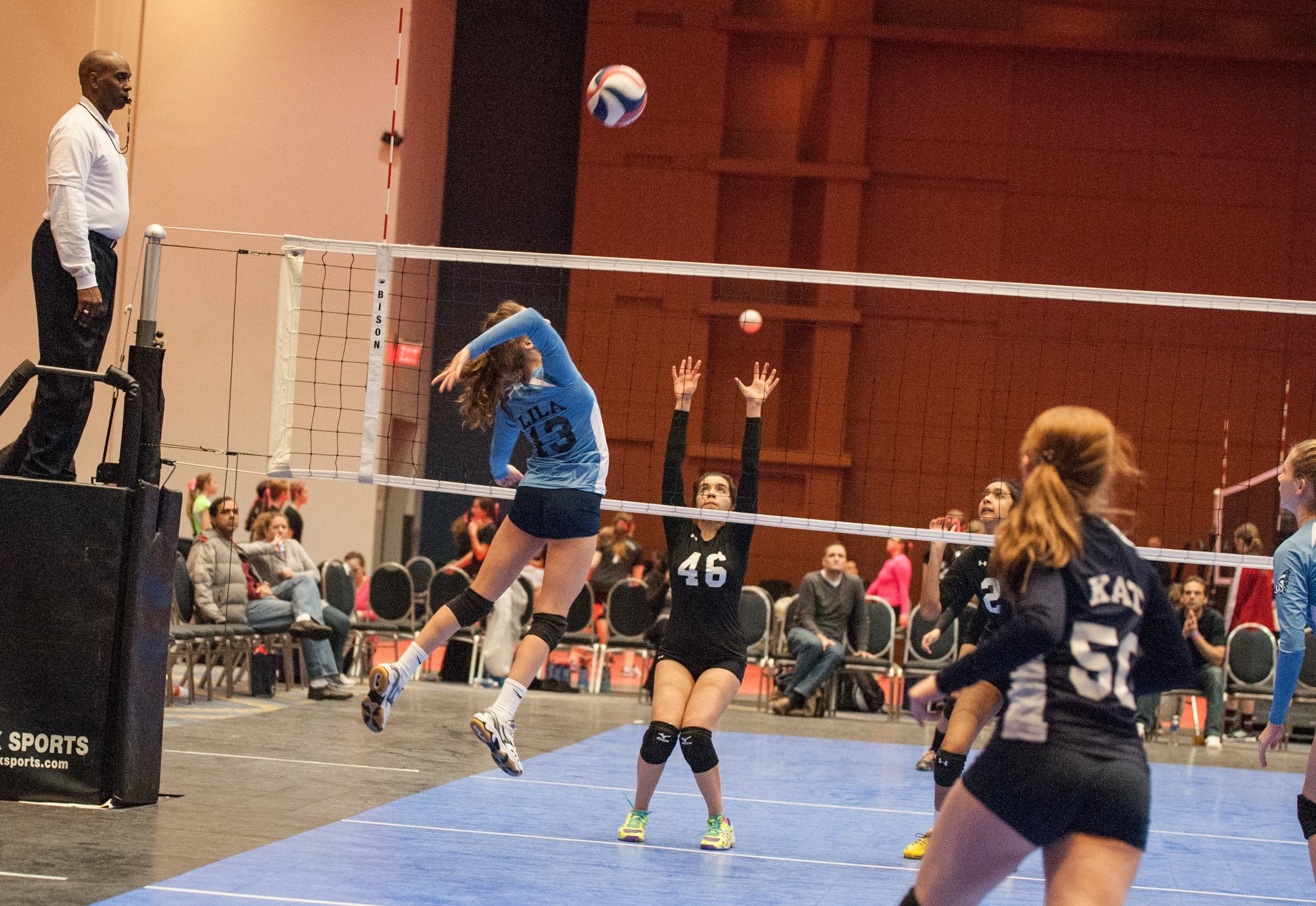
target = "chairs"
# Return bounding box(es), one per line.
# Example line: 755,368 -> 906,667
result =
1160,623 -> 1316,752
739,585 -> 959,724
321,556 -> 671,698
166,536 -> 307,707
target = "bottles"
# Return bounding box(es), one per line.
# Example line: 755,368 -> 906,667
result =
1168,715 -> 1179,746
547,663 -> 571,685
577,665 -> 587,691
172,685 -> 188,698
600,666 -> 610,693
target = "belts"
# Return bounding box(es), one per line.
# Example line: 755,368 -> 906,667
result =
89,230 -> 118,249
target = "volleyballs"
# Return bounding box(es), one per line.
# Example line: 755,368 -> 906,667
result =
585,64 -> 648,129
739,309 -> 763,334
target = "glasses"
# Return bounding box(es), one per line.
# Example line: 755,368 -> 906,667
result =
218,508 -> 240,515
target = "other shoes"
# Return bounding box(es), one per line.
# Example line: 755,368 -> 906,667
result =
771,694 -> 792,716
1205,735 -> 1223,747
1136,721 -> 1146,740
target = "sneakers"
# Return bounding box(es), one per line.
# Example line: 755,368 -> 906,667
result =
471,709 -> 525,776
700,812 -> 736,850
289,621 -> 333,641
308,683 -> 354,701
618,792 -> 655,843
362,662 -> 405,734
902,828 -> 932,860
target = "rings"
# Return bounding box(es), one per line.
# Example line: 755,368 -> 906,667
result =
84,309 -> 89,314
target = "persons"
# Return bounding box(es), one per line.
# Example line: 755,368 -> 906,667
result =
0,51 -> 134,479
101,302 -> 1316,905
